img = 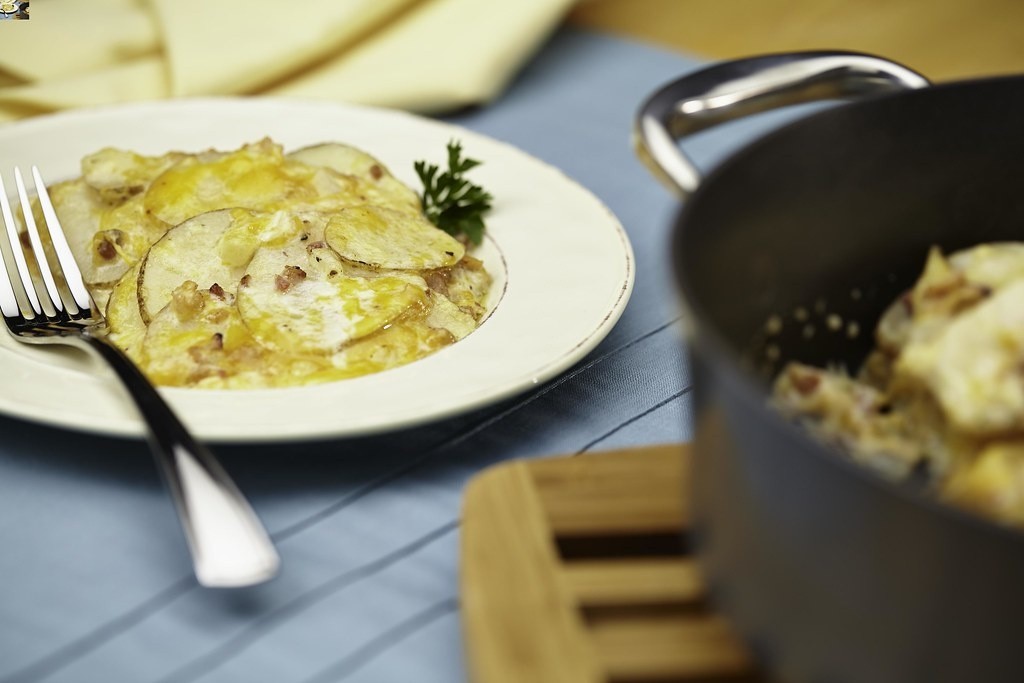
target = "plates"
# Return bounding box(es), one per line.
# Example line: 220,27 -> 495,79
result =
0,96 -> 636,444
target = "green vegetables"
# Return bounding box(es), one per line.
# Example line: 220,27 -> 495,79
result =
414,138 -> 491,247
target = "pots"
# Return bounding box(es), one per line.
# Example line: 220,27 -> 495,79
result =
633,49 -> 1024,683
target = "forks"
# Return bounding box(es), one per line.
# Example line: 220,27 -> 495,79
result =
0,165 -> 280,589
